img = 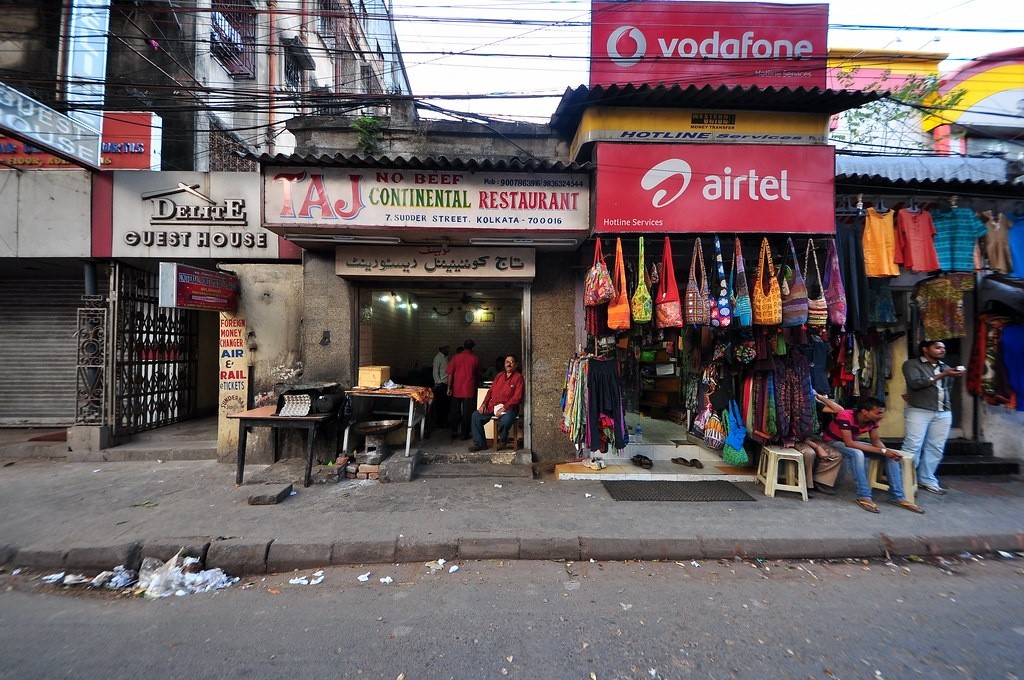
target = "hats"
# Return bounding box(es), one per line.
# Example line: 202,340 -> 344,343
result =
438,341 -> 450,349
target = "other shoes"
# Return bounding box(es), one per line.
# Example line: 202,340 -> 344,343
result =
632,455 -> 654,469
808,484 -> 835,497
468,444 -> 488,453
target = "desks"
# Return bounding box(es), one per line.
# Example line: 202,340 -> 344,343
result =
342,386 -> 432,458
226,404 -> 336,487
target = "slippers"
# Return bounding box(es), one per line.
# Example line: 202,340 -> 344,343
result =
888,498 -> 925,514
854,497 -> 880,514
671,458 -> 691,467
690,458 -> 703,469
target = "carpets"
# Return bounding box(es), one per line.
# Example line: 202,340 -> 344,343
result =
601,480 -> 757,502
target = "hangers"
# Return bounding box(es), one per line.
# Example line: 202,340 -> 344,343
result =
835,194 -> 1024,220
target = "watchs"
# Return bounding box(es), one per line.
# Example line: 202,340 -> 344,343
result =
881,448 -> 887,455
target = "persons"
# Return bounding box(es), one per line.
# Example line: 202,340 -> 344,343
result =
820,396 -> 926,514
469,353 -> 524,453
788,387 -> 846,498
901,338 -> 965,494
429,339 -> 504,442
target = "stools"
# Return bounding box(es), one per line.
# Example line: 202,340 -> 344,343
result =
867,447 -> 918,505
756,443 -> 809,501
491,412 -> 521,451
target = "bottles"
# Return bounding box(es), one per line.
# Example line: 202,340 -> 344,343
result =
635,424 -> 642,443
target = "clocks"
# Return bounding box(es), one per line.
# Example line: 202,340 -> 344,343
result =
464,311 -> 474,324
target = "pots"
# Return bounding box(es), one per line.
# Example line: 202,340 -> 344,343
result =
351,416 -> 404,435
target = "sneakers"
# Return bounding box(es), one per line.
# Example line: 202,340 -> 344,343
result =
918,484 -> 947,495
595,456 -> 606,468
582,457 -> 600,470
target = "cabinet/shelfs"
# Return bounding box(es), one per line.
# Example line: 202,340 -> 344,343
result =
638,321 -> 682,422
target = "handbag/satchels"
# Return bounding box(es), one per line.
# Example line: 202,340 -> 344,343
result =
585,235 -> 847,328
